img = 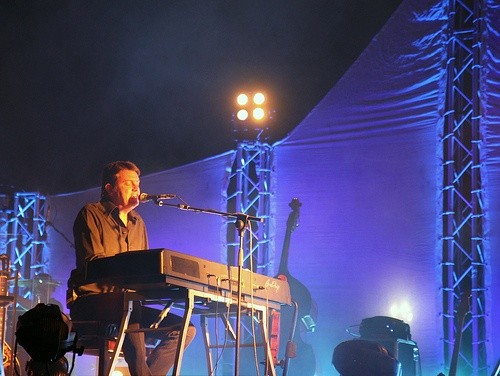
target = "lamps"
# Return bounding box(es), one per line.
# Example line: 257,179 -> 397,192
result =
14,302 -> 73,360
358,316 -> 412,360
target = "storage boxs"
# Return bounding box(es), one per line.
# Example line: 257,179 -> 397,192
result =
367,338 -> 419,376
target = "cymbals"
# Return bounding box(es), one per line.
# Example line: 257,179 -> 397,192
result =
13,278 -> 62,288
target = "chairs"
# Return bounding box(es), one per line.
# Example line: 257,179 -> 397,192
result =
71,316 -> 156,376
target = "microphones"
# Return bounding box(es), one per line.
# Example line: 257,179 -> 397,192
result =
138,192 -> 178,204
41,206 -> 51,241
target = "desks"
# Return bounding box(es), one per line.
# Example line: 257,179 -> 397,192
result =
79,275 -> 281,376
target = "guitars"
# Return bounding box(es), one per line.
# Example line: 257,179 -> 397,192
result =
239,196 -> 319,376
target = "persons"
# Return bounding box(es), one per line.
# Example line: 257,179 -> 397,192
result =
73,160 -> 197,376
332,340 -> 401,376
32,273 -> 64,313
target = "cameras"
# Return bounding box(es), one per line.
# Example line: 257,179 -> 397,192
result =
15,303 -> 73,376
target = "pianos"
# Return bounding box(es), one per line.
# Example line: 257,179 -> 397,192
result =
76,248 -> 292,317
